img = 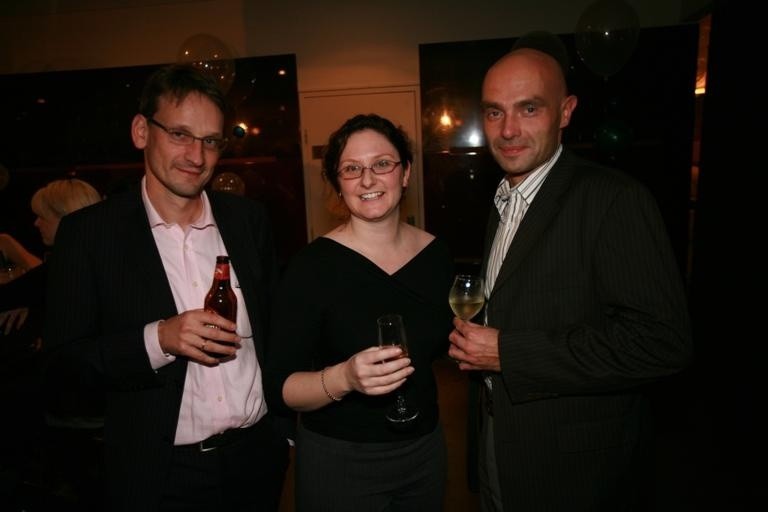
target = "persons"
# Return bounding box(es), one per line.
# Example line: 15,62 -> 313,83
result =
26,71 -> 287,511
444,48 -> 698,511
260,114 -> 466,511
0,179 -> 101,314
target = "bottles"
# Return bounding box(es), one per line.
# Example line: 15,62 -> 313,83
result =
205,254 -> 237,361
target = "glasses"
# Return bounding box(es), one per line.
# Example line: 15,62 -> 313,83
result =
149,117 -> 228,153
335,156 -> 403,182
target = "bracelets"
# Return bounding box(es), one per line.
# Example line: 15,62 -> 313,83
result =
322,367 -> 344,402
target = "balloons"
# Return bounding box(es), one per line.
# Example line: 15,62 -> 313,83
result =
179,35 -> 238,101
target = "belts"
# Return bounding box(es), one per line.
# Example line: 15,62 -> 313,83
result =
169,412 -> 271,464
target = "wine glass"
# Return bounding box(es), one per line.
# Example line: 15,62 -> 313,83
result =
377,314 -> 420,421
449,274 -> 484,363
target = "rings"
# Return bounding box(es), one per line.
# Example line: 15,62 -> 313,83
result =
200,339 -> 208,350
